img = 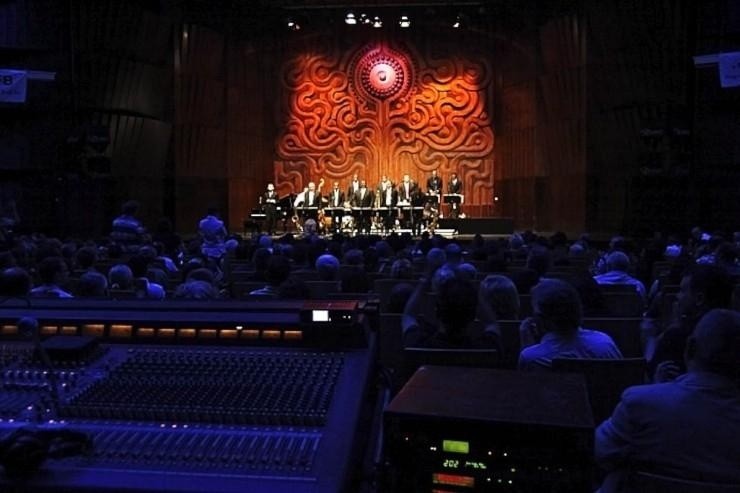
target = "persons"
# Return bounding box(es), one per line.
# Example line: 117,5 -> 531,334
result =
260,168 -> 462,236
2,200 -> 391,310
379,227 -> 740,492
